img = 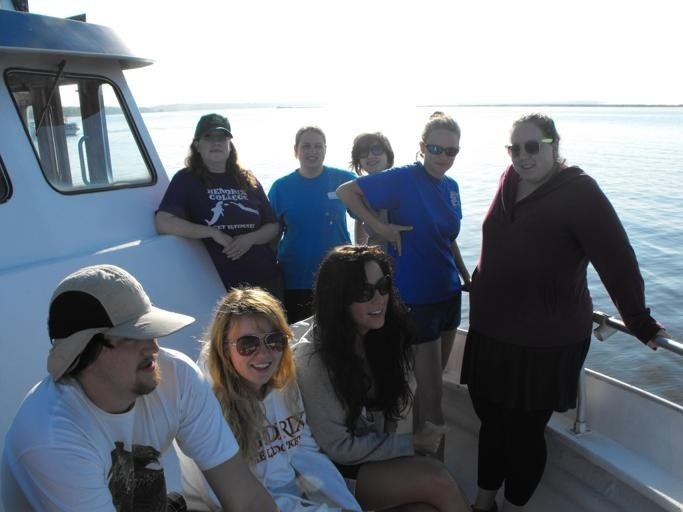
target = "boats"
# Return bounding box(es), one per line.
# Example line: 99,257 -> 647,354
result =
0,0 -> 682,509
27,115 -> 80,138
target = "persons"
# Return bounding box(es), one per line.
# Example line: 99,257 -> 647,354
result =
0,263 -> 282,512
170,284 -> 362,512
351,132 -> 391,246
291,242 -> 468,511
152,112 -> 282,312
458,113 -> 671,510
268,125 -> 368,319
336,111 -> 471,462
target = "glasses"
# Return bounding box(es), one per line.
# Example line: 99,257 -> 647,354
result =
505,138 -> 554,158
423,140 -> 460,156
223,331 -> 289,357
357,144 -> 385,158
352,274 -> 392,303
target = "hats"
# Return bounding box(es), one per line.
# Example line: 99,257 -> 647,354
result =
194,113 -> 233,141
47,264 -> 196,380
